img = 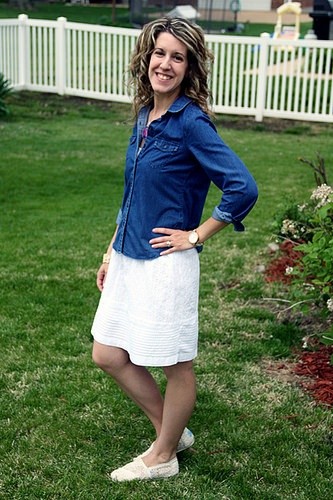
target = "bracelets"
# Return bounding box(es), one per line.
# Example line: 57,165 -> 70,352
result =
101,254 -> 111,264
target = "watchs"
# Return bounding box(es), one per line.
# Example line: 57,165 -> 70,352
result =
188,229 -> 204,247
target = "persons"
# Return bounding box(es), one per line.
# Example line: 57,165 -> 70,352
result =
89,16 -> 259,484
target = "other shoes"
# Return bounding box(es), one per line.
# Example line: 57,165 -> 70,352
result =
133,427 -> 195,462
111,454 -> 179,482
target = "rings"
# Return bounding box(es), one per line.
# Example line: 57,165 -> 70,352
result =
167,240 -> 171,248
